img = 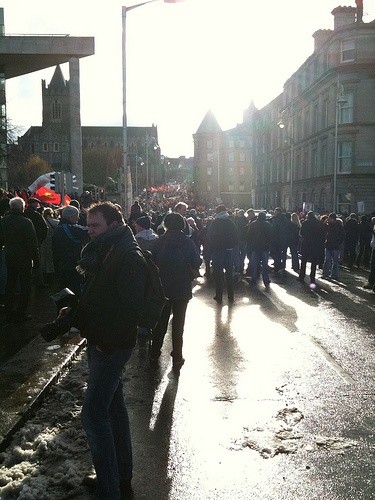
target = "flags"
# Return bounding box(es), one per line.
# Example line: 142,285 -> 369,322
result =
150,186 -> 166,193
35,183 -> 73,208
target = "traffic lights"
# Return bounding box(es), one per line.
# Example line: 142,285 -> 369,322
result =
50,172 -> 59,194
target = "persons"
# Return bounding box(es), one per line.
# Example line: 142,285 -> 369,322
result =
0,177 -> 375,320
148,212 -> 203,369
57,200 -> 148,500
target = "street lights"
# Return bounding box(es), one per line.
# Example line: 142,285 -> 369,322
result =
163,156 -> 170,180
277,107 -> 295,209
135,155 -> 145,196
120,0 -> 183,208
331,76 -> 347,214
147,140 -> 160,187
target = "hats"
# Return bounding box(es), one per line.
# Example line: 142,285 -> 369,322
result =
29,198 -> 41,204
62,206 -> 78,218
136,216 -> 150,230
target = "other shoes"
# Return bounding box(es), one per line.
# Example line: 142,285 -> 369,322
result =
7,314 -> 16,323
173,359 -> 184,365
150,359 -> 158,363
311,279 -> 315,282
321,275 -> 328,279
295,278 -> 303,281
90,494 -> 103,500
363,283 -> 373,289
204,273 -> 210,276
138,328 -> 150,335
214,297 -> 222,301
0,305 -> 5,312
198,274 -> 203,277
24,312 -> 32,320
83,475 -> 97,487
331,275 -> 336,279
228,300 -> 233,303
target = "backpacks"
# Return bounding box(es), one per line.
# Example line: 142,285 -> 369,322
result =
118,246 -> 169,330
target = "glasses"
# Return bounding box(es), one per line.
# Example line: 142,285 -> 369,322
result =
86,220 -> 105,229
